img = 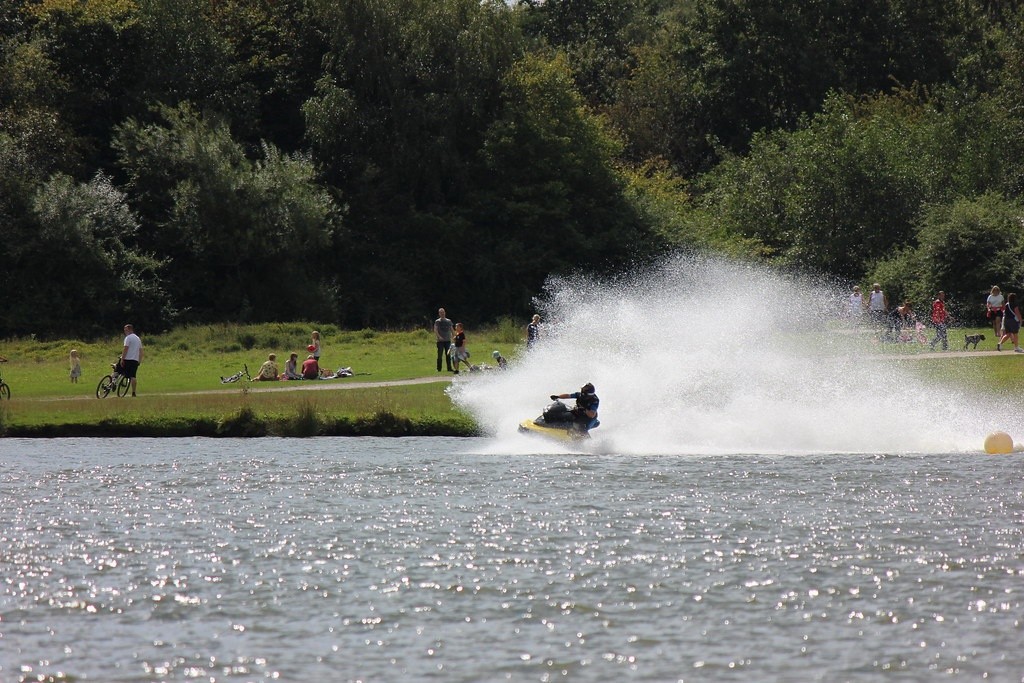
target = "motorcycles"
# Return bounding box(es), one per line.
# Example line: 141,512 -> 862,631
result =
516,399 -> 596,446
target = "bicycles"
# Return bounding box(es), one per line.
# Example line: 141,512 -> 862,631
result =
0,359 -> 11,401
220,362 -> 252,385
95,356 -> 134,400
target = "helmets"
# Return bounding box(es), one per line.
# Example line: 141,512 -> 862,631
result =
581,383 -> 595,400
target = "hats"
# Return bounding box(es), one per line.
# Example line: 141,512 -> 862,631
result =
492,351 -> 500,358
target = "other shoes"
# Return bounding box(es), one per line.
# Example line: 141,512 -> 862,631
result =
454,371 -> 459,374
448,369 -> 453,371
997,343 -> 1001,350
1015,348 -> 1023,352
438,369 -> 441,372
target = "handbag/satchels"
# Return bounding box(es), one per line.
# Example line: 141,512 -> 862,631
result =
1014,314 -> 1019,322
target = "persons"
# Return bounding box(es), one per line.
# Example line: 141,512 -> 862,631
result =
526,314 -> 541,349
882,301 -> 918,344
999,300 -> 1014,344
434,308 -> 456,371
110,324 -> 145,397
452,323 -> 475,373
928,291 -> 956,351
550,382 -> 599,439
986,286 -> 1005,337
848,286 -> 868,334
492,350 -> 507,371
250,354 -> 280,381
996,293 -> 1023,352
446,335 -> 460,372
306,330 -> 320,361
302,355 -> 319,379
285,352 -> 302,379
69,350 -> 81,384
867,283 -> 889,340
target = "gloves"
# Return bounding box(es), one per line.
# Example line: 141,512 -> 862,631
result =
550,395 -> 558,401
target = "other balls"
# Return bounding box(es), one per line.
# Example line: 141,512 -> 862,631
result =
308,345 -> 314,352
984,431 -> 1013,455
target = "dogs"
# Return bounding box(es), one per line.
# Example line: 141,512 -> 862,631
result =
963,334 -> 986,350
